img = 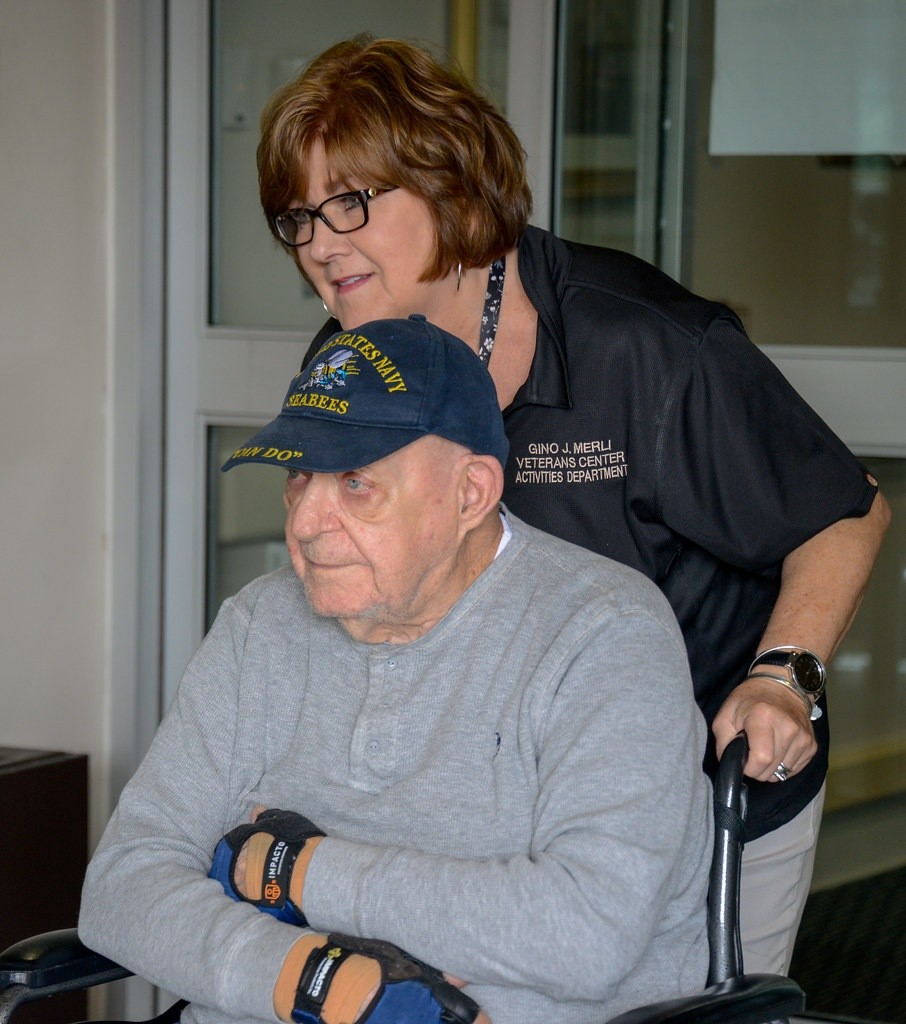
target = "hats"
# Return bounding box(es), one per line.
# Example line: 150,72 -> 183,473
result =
221,317 -> 515,476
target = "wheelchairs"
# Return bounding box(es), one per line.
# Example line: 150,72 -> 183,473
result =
0,715 -> 830,1024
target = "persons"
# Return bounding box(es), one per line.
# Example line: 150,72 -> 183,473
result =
76,314 -> 718,1024
254,30 -> 896,976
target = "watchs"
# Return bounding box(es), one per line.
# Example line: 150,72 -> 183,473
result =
744,646 -> 829,704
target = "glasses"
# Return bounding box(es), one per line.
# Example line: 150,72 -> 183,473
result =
271,180 -> 403,248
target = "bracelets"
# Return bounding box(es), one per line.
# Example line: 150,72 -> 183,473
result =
748,638 -> 827,723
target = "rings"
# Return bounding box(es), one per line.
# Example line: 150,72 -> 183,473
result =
772,762 -> 794,783
